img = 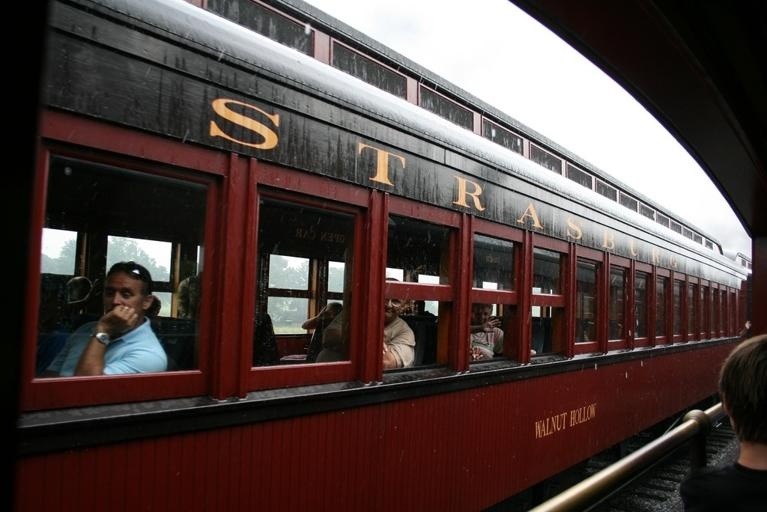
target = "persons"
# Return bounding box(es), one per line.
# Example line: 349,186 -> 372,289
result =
469,303 -> 504,361
402,298 -> 437,334
315,277 -> 416,369
301,302 -> 343,363
47,263 -> 167,376
253,286 -> 282,367
145,296 -> 162,333
679,335 -> 767,512
176,276 -> 199,320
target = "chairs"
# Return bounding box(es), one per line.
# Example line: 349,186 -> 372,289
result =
35,273 -> 550,369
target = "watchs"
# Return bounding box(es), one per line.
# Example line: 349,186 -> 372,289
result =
90,333 -> 110,346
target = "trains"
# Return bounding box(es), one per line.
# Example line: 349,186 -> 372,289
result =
1,0 -> 759,507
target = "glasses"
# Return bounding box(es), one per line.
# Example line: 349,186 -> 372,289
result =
106,263 -> 148,281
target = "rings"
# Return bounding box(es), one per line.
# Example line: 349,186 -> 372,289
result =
123,306 -> 129,310
